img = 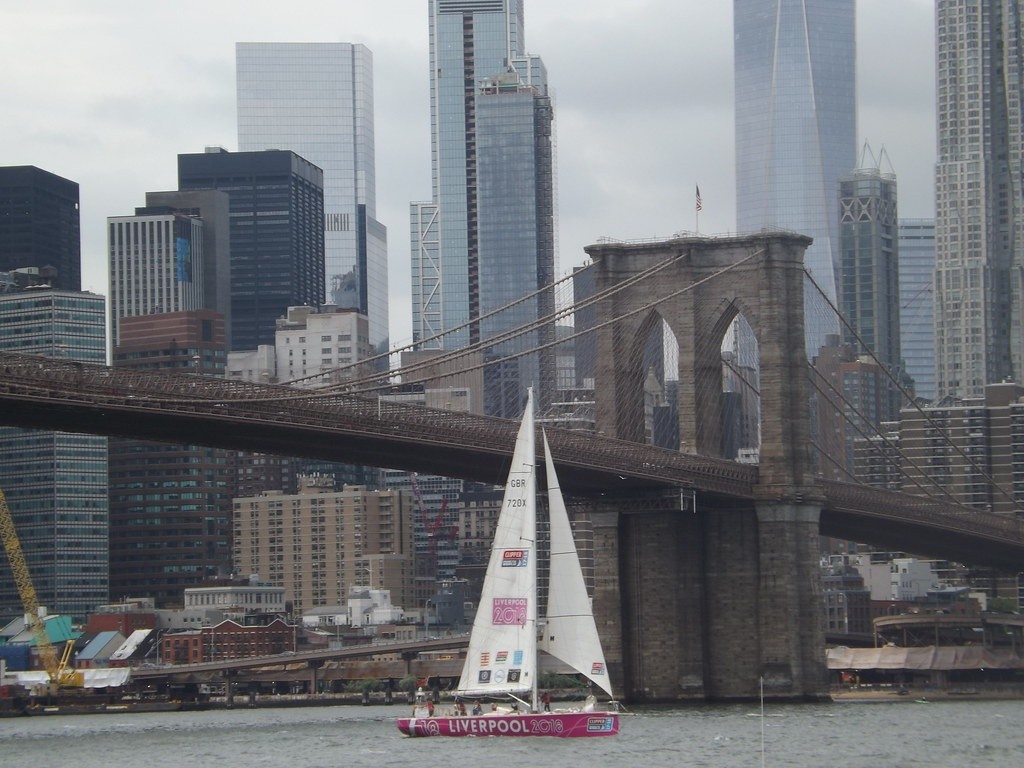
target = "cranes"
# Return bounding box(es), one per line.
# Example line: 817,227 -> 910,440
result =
0,488 -> 85,696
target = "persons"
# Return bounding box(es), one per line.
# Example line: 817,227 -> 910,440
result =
511,698 -> 519,714
459,700 -> 466,716
427,698 -> 434,717
490,703 -> 497,713
541,689 -> 551,712
473,700 -> 482,714
454,704 -> 458,716
413,696 -> 421,717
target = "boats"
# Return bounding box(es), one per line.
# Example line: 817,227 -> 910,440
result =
825,573 -> 1024,701
24,683 -> 182,715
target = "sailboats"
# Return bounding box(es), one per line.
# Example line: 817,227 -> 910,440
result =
397,380 -> 619,737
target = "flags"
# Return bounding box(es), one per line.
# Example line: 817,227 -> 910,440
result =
696,186 -> 701,211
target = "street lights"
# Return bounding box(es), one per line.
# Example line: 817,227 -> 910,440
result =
425,598 -> 432,638
294,614 -> 300,653
156,629 -> 166,669
